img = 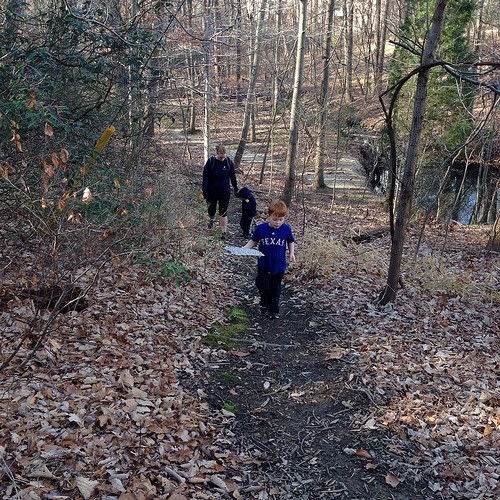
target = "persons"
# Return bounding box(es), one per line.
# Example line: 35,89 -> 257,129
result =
202,145 -> 238,239
237,187 -> 256,239
242,200 -> 296,318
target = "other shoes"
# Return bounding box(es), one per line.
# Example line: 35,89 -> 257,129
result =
259,306 -> 268,316
244,233 -> 251,238
208,219 -> 214,229
221,232 -> 227,239
269,313 -> 280,319
239,233 -> 245,237
